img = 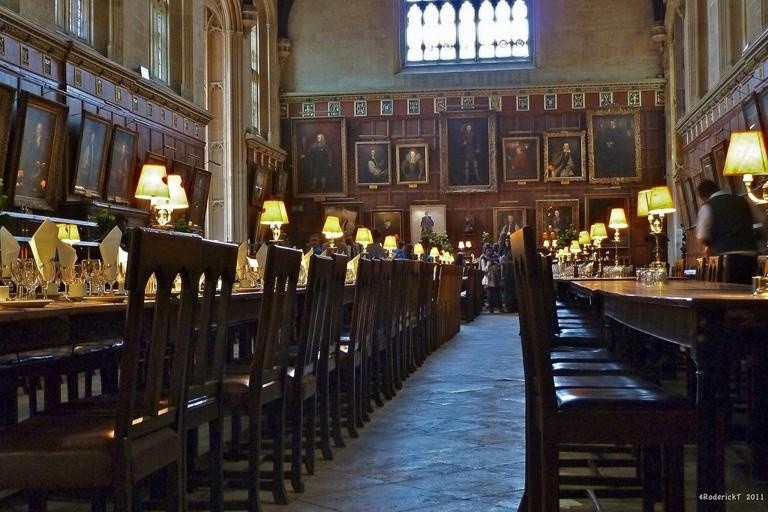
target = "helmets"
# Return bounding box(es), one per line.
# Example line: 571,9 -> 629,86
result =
483,243 -> 494,253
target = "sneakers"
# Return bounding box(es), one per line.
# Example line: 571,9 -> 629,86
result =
489,309 -> 494,313
499,308 -> 504,312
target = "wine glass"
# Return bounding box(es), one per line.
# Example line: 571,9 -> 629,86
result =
38,259 -> 54,301
12,258 -> 32,298
655,267 -> 666,284
105,263 -> 119,296
59,263 -> 71,300
71,265 -> 86,286
26,269 -> 40,298
82,259 -> 102,297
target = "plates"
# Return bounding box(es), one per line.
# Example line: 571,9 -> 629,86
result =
146,291 -> 180,300
84,295 -> 127,302
0,299 -> 53,308
233,286 -> 263,294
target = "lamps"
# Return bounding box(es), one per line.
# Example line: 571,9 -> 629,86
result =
723,131 -> 768,209
637,186 -> 677,281
260,200 -> 289,242
608,208 -> 628,266
321,216 -> 343,255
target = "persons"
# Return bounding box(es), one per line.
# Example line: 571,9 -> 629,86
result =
420,211 -> 434,237
695,178 -> 758,285
301,118 -> 629,191
114,214 -> 132,250
117,142 -> 130,195
305,225 -> 414,262
500,215 -> 519,234
83,130 -> 96,183
551,210 -> 563,228
481,237 -> 515,315
26,123 -> 47,163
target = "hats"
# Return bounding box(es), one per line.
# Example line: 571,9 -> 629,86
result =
505,235 -> 510,240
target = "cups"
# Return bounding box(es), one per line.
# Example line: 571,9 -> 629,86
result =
636,267 -> 655,285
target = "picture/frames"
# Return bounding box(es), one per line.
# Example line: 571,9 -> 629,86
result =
249,163 -> 271,209
741,91 -> 765,132
675,138 -> 750,231
0,81 -> 212,235
